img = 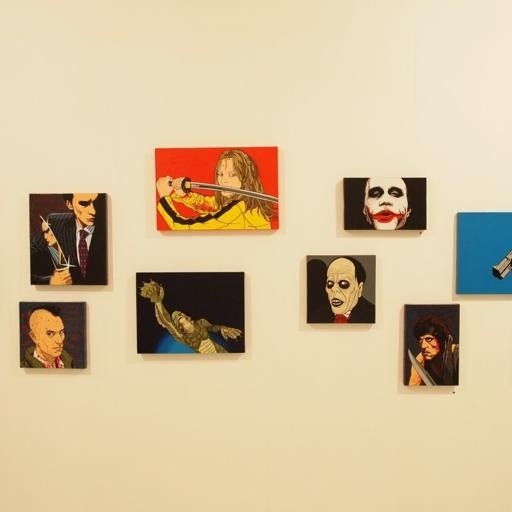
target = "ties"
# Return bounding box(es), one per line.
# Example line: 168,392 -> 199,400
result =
77,229 -> 91,279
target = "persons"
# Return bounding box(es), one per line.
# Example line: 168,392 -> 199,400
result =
324,255 -> 375,322
18,303 -> 84,368
141,279 -> 244,355
28,192 -> 108,285
359,177 -> 415,231
405,314 -> 459,386
154,147 -> 278,230
